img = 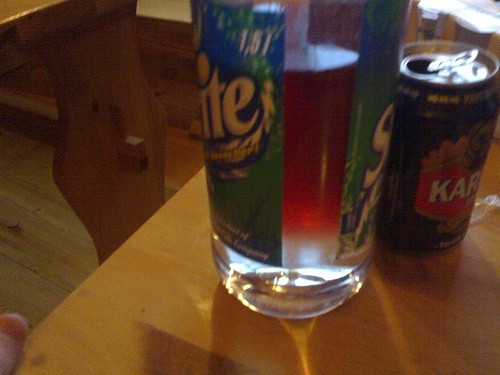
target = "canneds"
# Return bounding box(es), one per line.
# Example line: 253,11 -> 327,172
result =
374,39 -> 500,251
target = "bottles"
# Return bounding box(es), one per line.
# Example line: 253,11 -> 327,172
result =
189,0 -> 414,321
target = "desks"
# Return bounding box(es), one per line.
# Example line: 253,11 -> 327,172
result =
2,138 -> 500,375
